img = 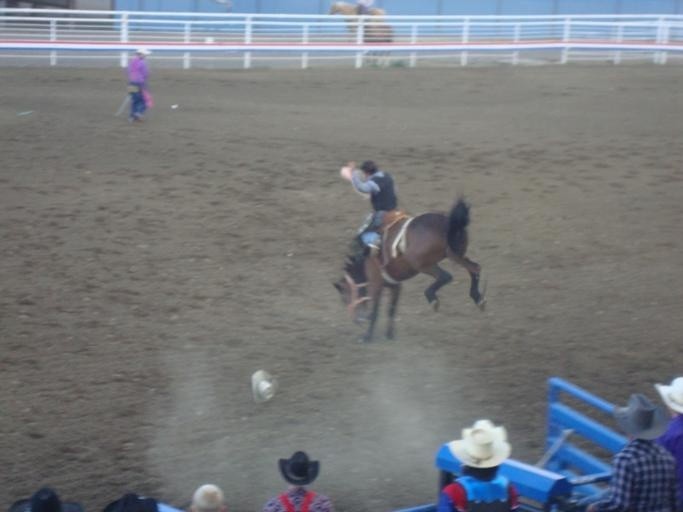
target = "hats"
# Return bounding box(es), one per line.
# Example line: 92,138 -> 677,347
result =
249,369 -> 280,405
612,392 -> 669,441
461,419 -> 507,442
192,483 -> 223,509
136,46 -> 152,56
7,487 -> 82,512
655,374 -> 683,414
277,451 -> 320,486
102,492 -> 158,512
446,428 -> 513,468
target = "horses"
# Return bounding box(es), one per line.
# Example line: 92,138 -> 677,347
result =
331,196 -> 490,345
332,1 -> 390,43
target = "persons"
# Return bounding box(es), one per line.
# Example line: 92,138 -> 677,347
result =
436,420 -> 520,511
653,377 -> 683,507
128,47 -> 154,123
189,483 -> 227,512
339,158 -> 398,255
263,451 -> 336,512
357,0 -> 372,16
587,393 -> 682,512
